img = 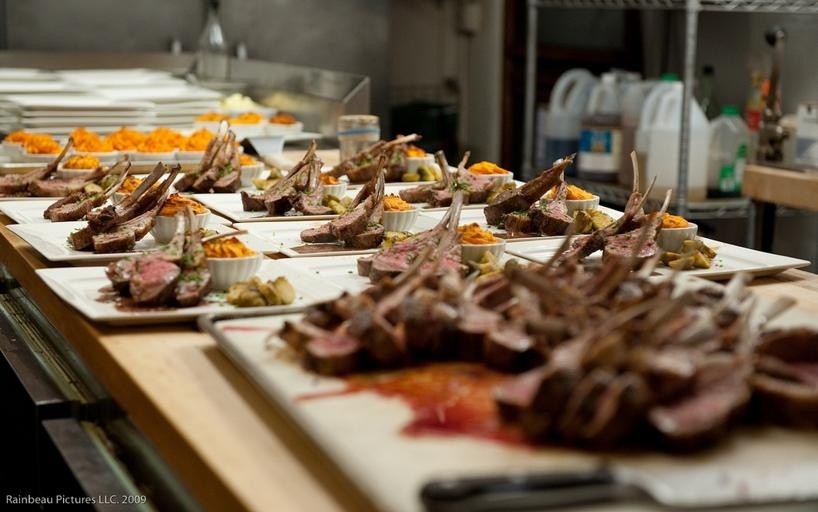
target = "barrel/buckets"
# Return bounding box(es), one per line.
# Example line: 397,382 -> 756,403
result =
539,70 -> 750,202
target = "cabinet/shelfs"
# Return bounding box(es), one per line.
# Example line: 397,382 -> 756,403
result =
519,3 -> 818,256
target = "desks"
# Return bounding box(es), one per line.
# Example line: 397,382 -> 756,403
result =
739,156 -> 818,257
